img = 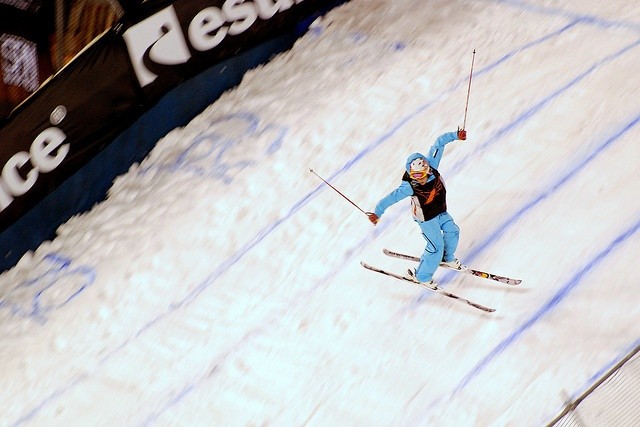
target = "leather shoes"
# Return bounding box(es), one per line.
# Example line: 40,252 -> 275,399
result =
458,126 -> 466,140
368,213 -> 378,223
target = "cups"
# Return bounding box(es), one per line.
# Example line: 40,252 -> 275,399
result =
409,170 -> 428,180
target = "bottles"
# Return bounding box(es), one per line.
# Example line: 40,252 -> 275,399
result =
409,158 -> 433,174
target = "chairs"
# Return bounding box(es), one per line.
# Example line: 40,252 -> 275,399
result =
416,273 -> 438,289
440,259 -> 465,269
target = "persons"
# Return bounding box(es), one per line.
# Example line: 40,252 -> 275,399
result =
369,130 -> 467,292
